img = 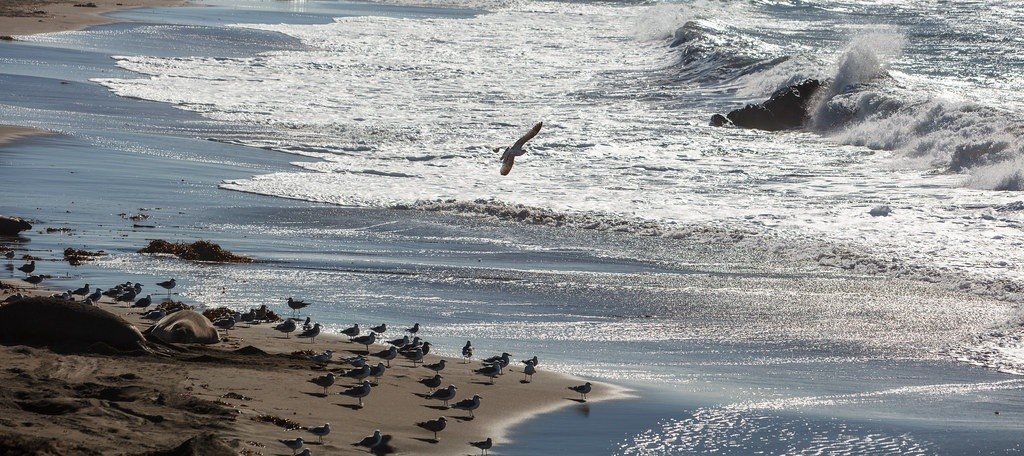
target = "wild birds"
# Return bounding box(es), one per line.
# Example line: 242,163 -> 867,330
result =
450,394 -> 484,418
278,437 -> 304,456
469,437 -> 492,456
307,373 -> 336,394
418,374 -> 443,390
350,430 -> 383,453
5,250 -> 36,276
568,382 -> 592,400
339,364 -> 370,383
339,380 -> 372,406
295,448 -> 312,456
54,278 -> 176,323
414,416 -> 448,439
211,296 -> 539,384
493,122 -> 543,175
305,423 -> 330,444
430,384 -> 459,407
369,362 -> 385,383
21,274 -> 46,289
